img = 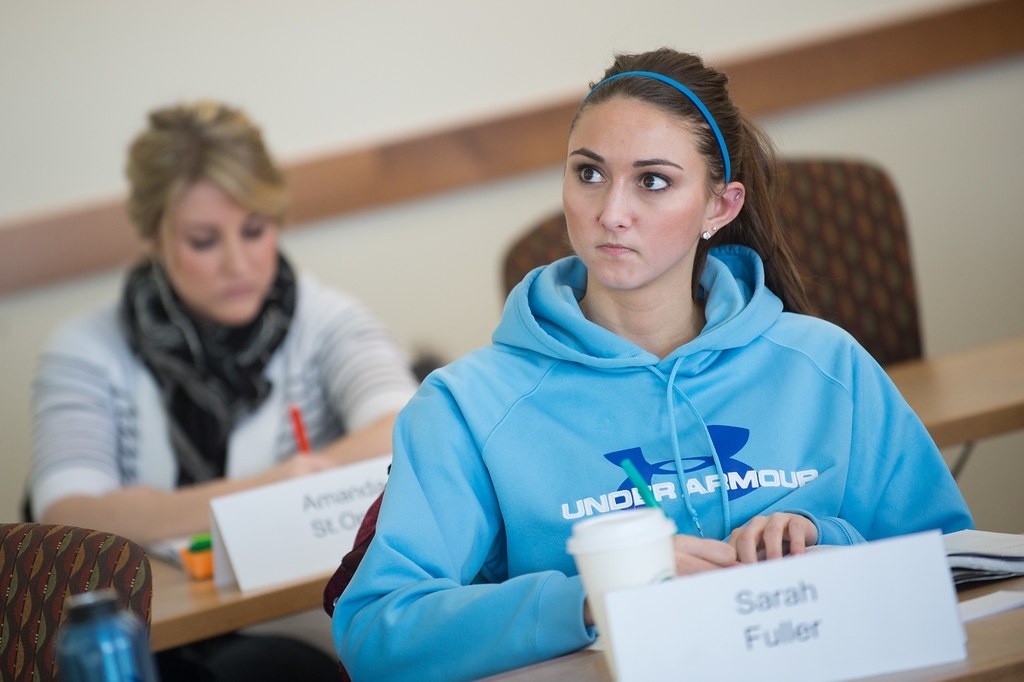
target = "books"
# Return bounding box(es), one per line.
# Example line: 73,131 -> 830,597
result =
940,528 -> 1024,587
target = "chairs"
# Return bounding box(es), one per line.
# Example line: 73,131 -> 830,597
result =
505,159 -> 923,381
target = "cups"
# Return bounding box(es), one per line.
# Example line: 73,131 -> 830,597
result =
564,506 -> 677,682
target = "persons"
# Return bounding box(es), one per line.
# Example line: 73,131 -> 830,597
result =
20,99 -> 424,682
324,48 -> 974,681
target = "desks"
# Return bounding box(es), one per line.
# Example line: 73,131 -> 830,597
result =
32,337 -> 1024,682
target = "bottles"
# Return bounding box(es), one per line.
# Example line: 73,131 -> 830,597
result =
53,585 -> 153,682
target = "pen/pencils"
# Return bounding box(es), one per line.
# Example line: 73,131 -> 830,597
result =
620,457 -> 659,509
290,407 -> 308,452
